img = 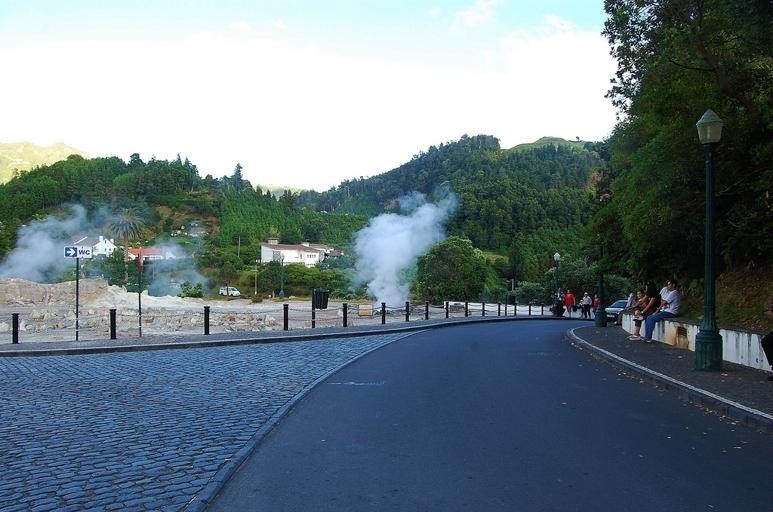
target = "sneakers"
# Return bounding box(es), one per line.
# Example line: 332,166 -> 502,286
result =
629,335 -> 652,343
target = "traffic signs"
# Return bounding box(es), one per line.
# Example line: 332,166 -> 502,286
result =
64,246 -> 93,258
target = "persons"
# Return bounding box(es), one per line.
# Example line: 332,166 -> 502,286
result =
553,287 -> 600,319
614,280 -> 681,343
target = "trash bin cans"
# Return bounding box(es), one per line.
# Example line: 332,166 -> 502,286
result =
315,289 -> 329,309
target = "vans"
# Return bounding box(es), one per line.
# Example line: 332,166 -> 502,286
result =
219,286 -> 241,297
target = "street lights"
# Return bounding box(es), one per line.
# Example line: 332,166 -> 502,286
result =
595,231 -> 607,327
695,107 -> 724,372
279,254 -> 285,298
554,253 -> 560,306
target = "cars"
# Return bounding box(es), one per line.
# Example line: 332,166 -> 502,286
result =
605,299 -> 636,317
450,303 -> 466,310
